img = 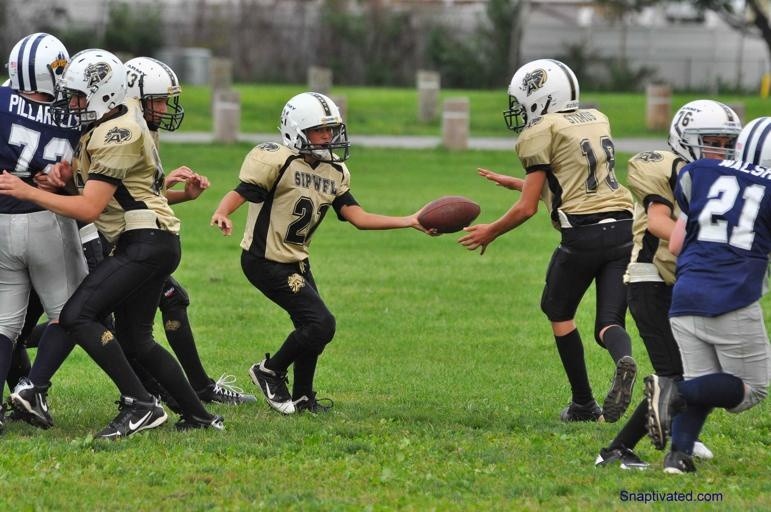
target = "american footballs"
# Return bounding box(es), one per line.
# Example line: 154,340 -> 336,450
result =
416,196 -> 480,232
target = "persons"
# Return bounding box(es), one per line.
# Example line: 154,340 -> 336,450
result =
121,55 -> 259,407
0,32 -> 89,436
594,100 -> 744,475
0,48 -> 228,445
209,92 -> 442,416
455,60 -> 637,422
4,82 -> 105,416
642,117 -> 771,476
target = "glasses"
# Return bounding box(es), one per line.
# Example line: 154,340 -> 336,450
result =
704,139 -> 734,150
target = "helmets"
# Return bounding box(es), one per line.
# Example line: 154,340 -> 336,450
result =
504,59 -> 580,134
8,33 -> 69,98
281,92 -> 352,164
50,50 -> 128,126
734,117 -> 771,170
125,56 -> 184,131
669,100 -> 742,164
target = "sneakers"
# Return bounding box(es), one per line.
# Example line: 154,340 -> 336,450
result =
594,445 -> 652,471
0,402 -> 7,432
603,355 -> 637,423
94,395 -> 169,440
294,391 -> 335,416
691,439 -> 713,460
663,452 -> 697,475
250,353 -> 296,415
643,373 -> 684,450
171,415 -> 226,432
195,372 -> 258,405
561,402 -> 602,424
8,376 -> 54,429
